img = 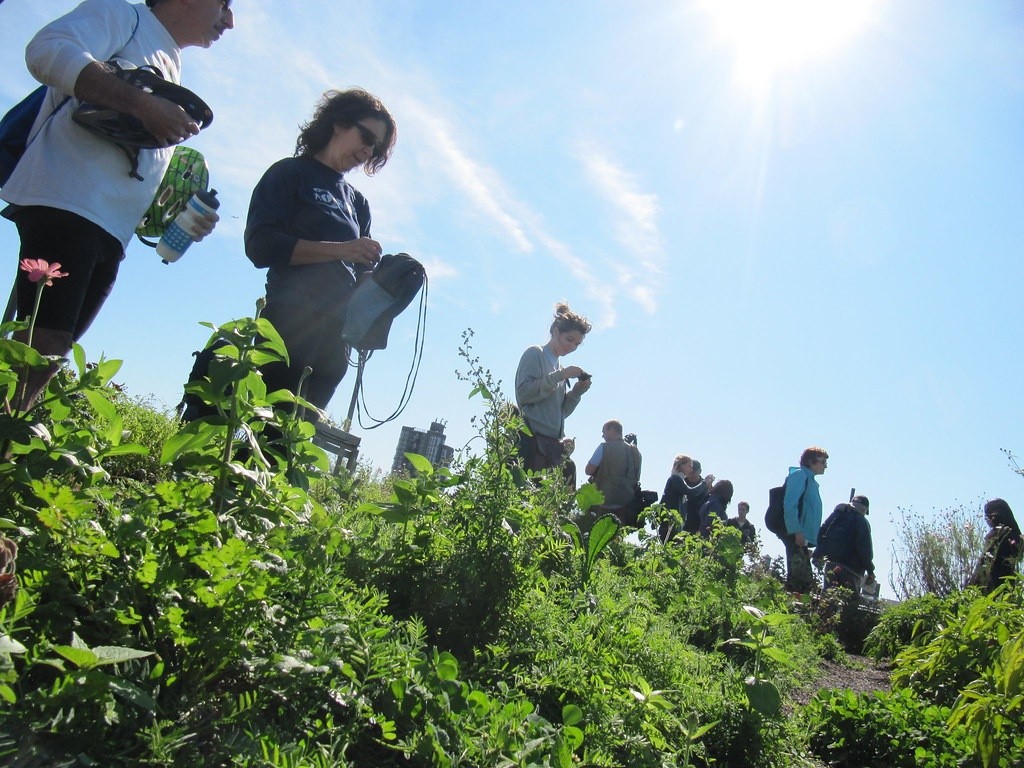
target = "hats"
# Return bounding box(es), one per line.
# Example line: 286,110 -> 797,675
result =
692,461 -> 701,470
851,495 -> 869,515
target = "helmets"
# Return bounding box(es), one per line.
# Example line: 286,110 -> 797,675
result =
72,54 -> 213,150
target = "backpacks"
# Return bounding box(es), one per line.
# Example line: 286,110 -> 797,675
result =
0,4 -> 140,188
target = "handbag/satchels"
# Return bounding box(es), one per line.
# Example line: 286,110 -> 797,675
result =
527,431 -> 564,473
341,252 -> 428,429
625,487 -> 646,526
764,476 -> 808,535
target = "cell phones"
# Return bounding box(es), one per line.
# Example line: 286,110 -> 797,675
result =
578,372 -> 592,382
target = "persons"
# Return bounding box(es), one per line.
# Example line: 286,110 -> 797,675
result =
970,498 -> 1024,596
726,502 -> 756,546
499,403 -> 519,426
586,420 -> 642,525
243,90 -> 398,467
658,454 -> 733,548
812,495 -> 876,653
514,303 -> 592,484
561,439 -> 576,491
783,447 -> 829,594
0,0 -> 235,436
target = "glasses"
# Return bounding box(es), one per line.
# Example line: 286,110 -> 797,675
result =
817,460 -> 826,465
224,0 -> 233,10
984,514 -> 996,520
354,122 -> 384,155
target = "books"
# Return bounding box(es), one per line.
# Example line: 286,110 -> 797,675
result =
862,583 -> 880,599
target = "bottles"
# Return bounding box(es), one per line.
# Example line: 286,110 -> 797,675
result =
156,187 -> 220,263
